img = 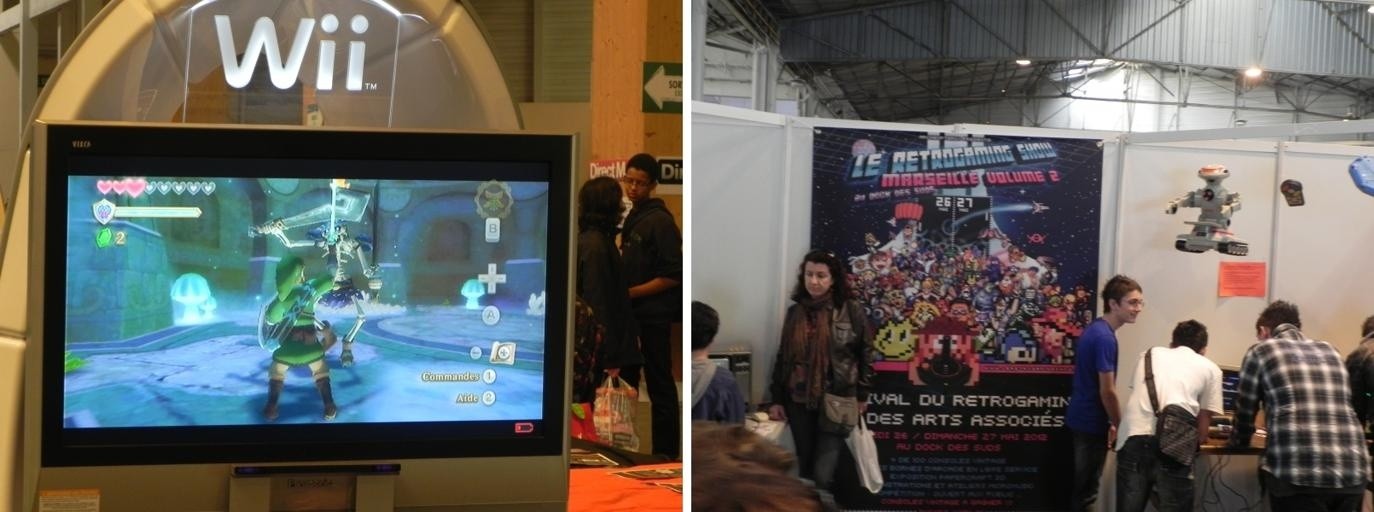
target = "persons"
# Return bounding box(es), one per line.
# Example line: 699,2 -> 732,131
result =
1165,165 -> 1248,256
263,244 -> 339,422
1064,274 -> 1143,512
577,153 -> 682,459
1219,275 -> 1374,512
848,225 -> 1092,386
1115,319 -> 1225,512
769,250 -> 873,489
691,301 -> 745,421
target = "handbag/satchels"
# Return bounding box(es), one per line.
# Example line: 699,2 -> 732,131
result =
817,393 -> 858,436
1154,404 -> 1200,470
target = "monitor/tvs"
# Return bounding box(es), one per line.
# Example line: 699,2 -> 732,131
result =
22,119 -> 582,512
1213,363 -> 1241,415
707,343 -> 753,417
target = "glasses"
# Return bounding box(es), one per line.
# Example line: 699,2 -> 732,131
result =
623,176 -> 654,187
1121,299 -> 1146,308
